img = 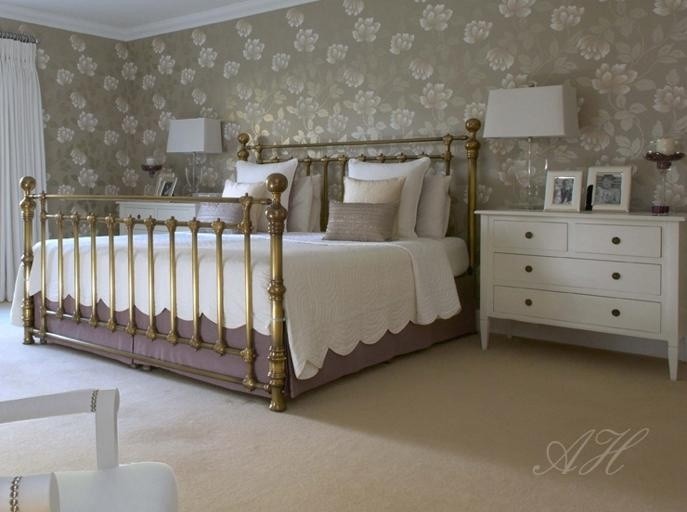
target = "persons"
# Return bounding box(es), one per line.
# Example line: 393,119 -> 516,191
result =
561,179 -> 573,204
596,174 -> 620,204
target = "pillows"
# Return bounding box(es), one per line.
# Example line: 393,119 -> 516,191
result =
195,152 -> 455,243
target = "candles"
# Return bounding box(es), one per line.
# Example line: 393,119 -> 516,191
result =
653,136 -> 676,157
145,158 -> 155,166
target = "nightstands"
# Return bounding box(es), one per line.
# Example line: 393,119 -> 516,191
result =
472,207 -> 685,381
114,201 -> 202,236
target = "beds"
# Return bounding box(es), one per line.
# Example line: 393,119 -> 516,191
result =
16,117 -> 486,413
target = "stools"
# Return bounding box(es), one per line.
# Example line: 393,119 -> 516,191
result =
1,388 -> 179,512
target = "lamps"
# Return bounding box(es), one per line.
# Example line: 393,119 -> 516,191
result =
480,80 -> 580,210
165,117 -> 222,196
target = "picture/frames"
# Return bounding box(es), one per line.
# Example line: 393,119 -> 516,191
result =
158,177 -> 178,197
542,165 -> 632,213
